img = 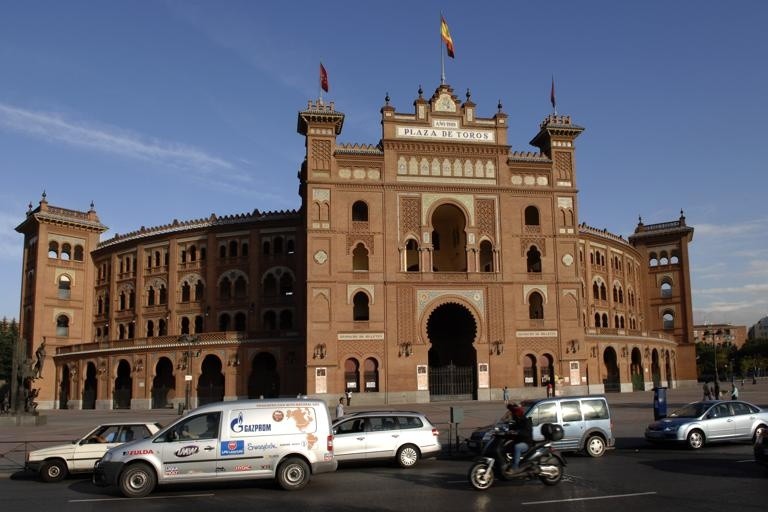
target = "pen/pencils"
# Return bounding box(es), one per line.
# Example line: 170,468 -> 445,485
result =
329,408 -> 441,469
92,398 -> 337,498
469,395 -> 615,458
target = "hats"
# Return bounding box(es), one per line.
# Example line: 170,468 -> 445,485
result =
644,399 -> 768,449
25,419 -> 162,482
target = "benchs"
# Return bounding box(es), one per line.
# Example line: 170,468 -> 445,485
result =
550,80 -> 556,108
319,63 -> 329,93
439,15 -> 455,60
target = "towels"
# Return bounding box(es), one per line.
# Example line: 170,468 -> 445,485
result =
179,403 -> 184,415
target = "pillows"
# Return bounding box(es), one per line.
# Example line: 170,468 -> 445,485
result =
512,407 -> 525,416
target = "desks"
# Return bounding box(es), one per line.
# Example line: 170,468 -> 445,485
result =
704,328 -> 724,400
177,332 -> 200,407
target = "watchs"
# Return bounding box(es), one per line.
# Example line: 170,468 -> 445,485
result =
468,422 -> 564,491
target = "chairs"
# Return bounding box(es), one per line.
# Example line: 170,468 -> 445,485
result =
504,468 -> 519,476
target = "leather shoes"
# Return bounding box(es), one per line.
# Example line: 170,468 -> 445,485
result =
506,430 -> 518,436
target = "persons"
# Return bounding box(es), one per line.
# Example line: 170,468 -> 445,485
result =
33,336 -> 48,380
0,390 -> 10,414
502,402 -> 533,474
336,397 -> 346,418
546,382 -> 553,398
345,389 -> 353,405
28,387 -> 41,409
702,377 -> 745,401
91,426 -> 122,444
500,402 -> 521,424
180,414 -> 218,440
503,386 -> 511,404
707,406 -> 718,419
